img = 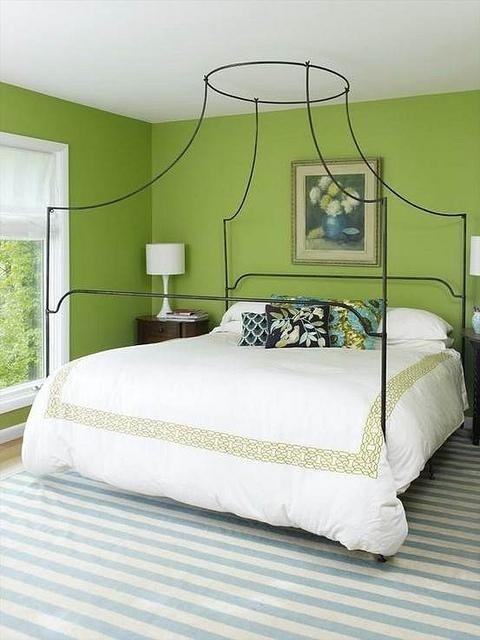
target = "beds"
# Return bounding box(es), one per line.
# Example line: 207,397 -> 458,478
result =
45,328 -> 466,561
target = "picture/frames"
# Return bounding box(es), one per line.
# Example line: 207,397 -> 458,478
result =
291,157 -> 382,267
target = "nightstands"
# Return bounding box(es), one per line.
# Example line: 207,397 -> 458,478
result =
135,315 -> 208,345
460,327 -> 480,446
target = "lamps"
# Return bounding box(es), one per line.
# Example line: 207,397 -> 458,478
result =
469,235 -> 480,334
145,243 -> 186,318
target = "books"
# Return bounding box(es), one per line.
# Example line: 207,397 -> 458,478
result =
164,306 -> 209,324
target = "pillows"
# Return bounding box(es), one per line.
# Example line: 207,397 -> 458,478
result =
209,294 -> 456,354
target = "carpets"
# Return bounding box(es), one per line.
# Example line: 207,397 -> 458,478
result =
0,429 -> 480,640
